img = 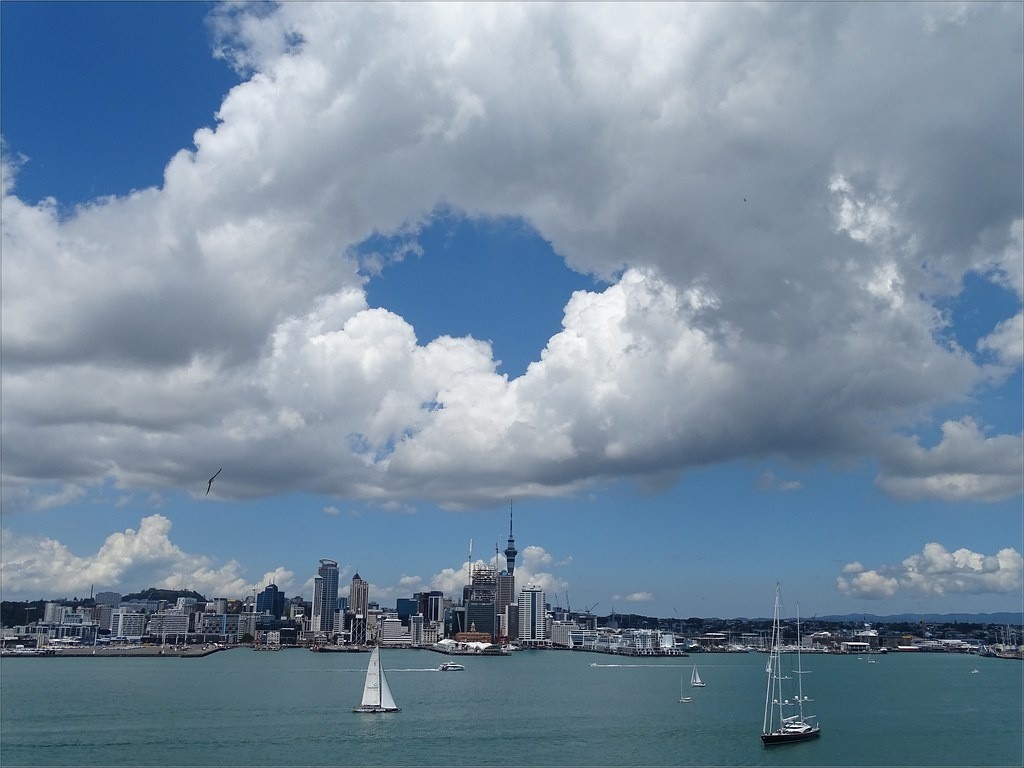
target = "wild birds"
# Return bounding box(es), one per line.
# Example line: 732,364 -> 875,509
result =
205,467 -> 223,496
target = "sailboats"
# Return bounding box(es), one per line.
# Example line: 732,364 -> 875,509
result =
691,664 -> 706,687
353,644 -> 402,713
764,657 -> 773,673
760,579 -> 821,746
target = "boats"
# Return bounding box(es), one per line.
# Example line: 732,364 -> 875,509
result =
678,674 -> 693,703
868,659 -> 879,664
439,661 -> 466,671
